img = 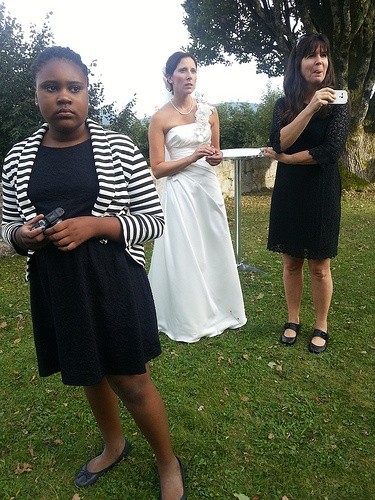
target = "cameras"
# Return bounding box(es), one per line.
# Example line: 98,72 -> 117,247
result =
30,207 -> 65,233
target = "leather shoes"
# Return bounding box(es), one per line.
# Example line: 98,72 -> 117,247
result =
310,330 -> 329,354
281,320 -> 301,345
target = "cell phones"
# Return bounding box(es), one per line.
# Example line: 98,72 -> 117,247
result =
327,90 -> 348,104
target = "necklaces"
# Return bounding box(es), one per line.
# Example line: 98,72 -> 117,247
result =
170,97 -> 194,115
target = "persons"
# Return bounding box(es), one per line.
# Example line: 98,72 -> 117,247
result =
0,46 -> 191,500
145,52 -> 223,340
267,33 -> 350,352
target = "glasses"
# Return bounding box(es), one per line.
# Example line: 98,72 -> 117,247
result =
296,32 -> 323,47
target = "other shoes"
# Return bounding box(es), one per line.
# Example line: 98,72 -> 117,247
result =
76,438 -> 128,487
159,456 -> 187,500
